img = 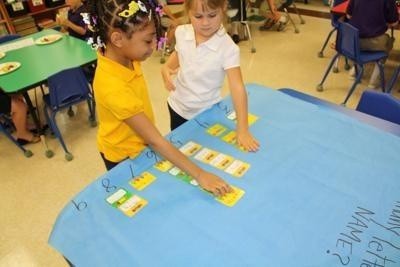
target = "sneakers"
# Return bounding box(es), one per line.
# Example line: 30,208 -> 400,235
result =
368,82 -> 381,88
349,69 -> 356,80
277,16 -> 290,31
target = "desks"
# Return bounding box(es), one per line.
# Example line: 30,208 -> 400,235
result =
48,83 -> 400,266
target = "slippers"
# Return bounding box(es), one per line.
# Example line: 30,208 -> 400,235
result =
17,134 -> 40,144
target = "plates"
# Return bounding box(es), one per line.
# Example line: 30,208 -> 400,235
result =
0,52 -> 21,76
33,34 -> 64,45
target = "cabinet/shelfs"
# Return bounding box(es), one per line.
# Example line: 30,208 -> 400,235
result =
0,0 -> 72,37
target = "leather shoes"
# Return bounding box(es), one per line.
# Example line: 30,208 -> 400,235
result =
277,0 -> 292,11
259,18 -> 279,30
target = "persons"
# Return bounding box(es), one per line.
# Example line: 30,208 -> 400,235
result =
0,90 -> 49,144
261,0 -> 290,31
347,0 -> 399,88
57,0 -> 88,39
162,0 -> 259,152
92,0 -> 234,196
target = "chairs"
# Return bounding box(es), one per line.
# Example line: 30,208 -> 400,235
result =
161,25 -> 169,64
231,1 -> 256,53
0,25 -> 97,160
278,0 -> 306,33
318,0 -> 400,124
